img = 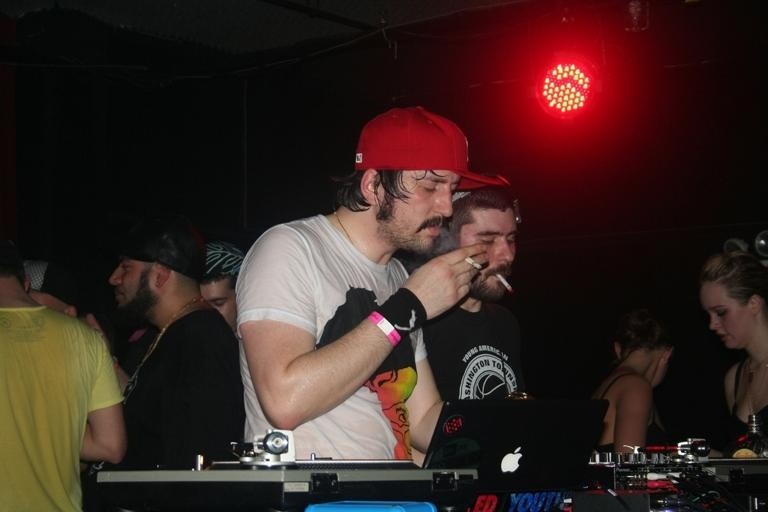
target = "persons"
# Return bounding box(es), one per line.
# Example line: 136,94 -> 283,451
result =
592,305 -> 677,454
692,250 -> 767,456
0,214 -> 246,511
394,183 -> 525,466
235,105 -> 495,460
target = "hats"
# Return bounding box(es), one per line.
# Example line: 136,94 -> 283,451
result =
353,104 -> 506,187
110,211 -> 244,278
23,254 -> 79,306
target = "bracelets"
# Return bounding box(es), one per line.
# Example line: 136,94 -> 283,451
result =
368,311 -> 401,347
377,287 -> 427,338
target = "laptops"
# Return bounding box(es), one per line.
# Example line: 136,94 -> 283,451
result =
422,399 -> 610,494
